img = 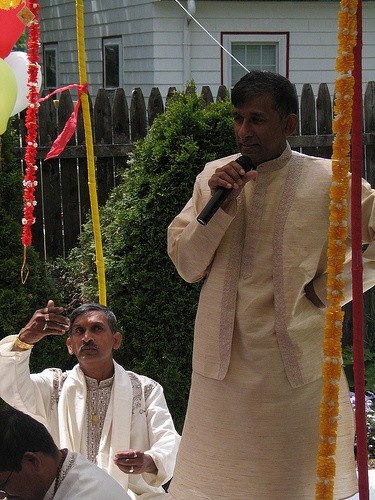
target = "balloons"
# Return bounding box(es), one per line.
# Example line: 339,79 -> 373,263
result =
2,1 -> 42,136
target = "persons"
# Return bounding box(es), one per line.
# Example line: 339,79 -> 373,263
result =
0,299 -> 180,499
1,398 -> 133,500
167,70 -> 374,500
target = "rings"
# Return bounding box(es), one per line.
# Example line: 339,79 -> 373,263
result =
133,451 -> 137,458
44,314 -> 49,321
129,466 -> 134,472
43,323 -> 48,330
43,308 -> 48,313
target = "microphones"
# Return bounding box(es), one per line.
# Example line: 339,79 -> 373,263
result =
196,155 -> 253,225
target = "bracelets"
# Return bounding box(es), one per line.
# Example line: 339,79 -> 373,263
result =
15,338 -> 34,350
